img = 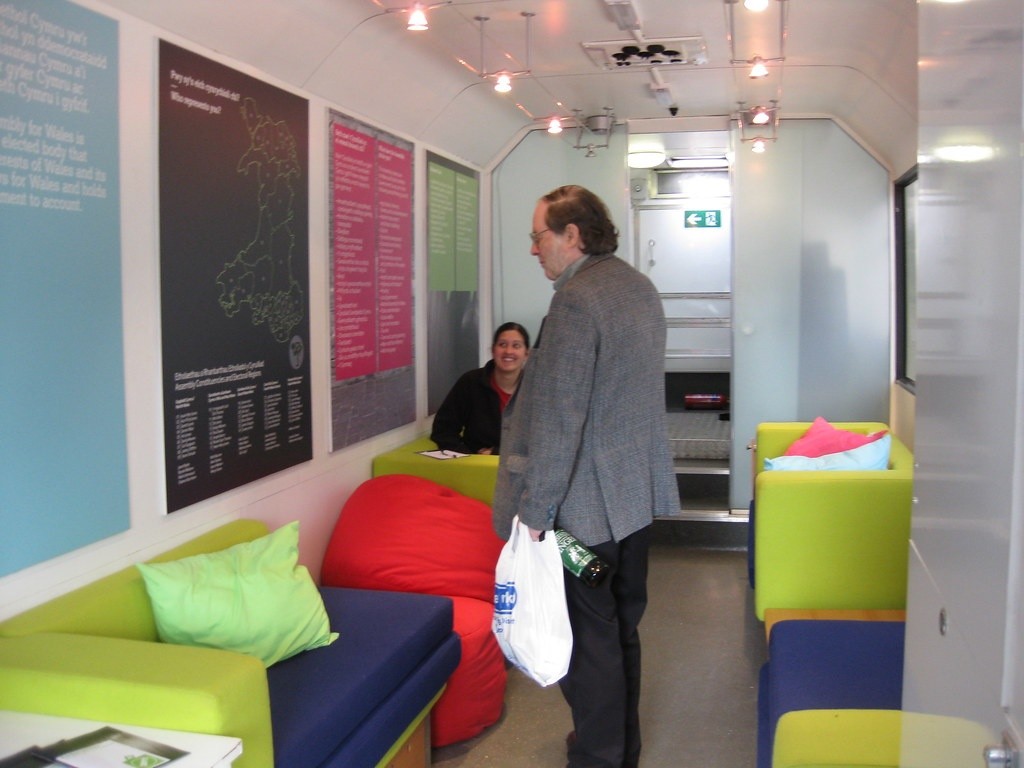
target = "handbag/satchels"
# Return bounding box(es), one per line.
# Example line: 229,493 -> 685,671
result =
490,511 -> 574,687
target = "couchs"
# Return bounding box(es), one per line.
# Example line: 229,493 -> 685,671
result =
746,421 -> 914,622
0,521 -> 463,768
757,619 -> 1001,768
371,433 -> 501,511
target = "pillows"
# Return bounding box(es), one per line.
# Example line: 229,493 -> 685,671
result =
763,434 -> 891,470
783,417 -> 889,459
133,521 -> 342,671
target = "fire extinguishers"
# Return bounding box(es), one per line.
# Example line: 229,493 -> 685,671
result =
684,393 -> 730,409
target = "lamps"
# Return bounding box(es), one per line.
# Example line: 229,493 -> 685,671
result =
482,69 -> 528,93
573,144 -> 609,158
534,116 -> 573,133
735,106 -> 780,125
731,56 -> 784,79
725,0 -> 769,12
741,136 -> 777,153
627,140 -> 667,168
386,1 -> 447,31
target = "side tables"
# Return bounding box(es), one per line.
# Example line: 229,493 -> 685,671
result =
0,710 -> 244,768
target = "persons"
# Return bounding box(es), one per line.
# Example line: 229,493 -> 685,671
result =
493,185 -> 680,768
432,323 -> 531,454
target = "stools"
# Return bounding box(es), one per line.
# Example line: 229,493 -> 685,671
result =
429,594 -> 508,747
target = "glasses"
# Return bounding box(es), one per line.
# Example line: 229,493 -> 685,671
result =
529,227 -> 551,244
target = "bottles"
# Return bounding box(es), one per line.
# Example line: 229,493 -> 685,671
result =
539,523 -> 610,588
